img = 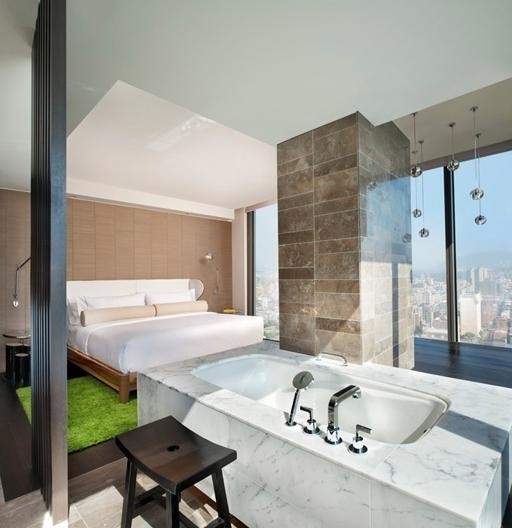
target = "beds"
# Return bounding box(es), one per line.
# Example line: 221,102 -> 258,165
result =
66,279 -> 264,403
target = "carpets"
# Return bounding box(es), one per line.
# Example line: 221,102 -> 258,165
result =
15,374 -> 137,453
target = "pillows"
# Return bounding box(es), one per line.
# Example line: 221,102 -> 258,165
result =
66,288 -> 208,327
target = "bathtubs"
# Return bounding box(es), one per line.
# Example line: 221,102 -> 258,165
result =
192,353 -> 449,445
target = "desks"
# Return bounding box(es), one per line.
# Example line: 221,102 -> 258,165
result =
2,330 -> 31,387
217,312 -> 245,315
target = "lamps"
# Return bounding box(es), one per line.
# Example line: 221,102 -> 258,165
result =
411,107 -> 486,237
403,217 -> 411,243
205,253 -> 220,295
12,257 -> 31,308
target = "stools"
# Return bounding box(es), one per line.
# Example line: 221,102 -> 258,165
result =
116,416 -> 236,528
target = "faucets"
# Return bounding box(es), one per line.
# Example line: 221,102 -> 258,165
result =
325,385 -> 362,444
